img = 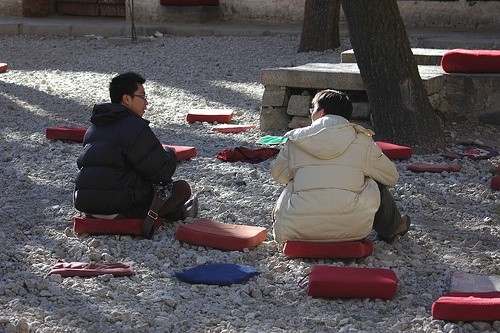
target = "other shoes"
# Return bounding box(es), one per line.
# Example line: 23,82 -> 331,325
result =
177,193 -> 199,221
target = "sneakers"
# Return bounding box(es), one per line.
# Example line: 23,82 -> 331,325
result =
376,214 -> 411,245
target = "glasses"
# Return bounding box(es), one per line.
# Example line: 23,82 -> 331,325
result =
121,93 -> 148,100
309,108 -> 321,114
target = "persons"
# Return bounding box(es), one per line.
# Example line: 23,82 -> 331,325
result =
270,89 -> 411,244
73,71 -> 198,220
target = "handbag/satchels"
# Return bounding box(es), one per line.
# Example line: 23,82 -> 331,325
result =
149,176 -> 174,202
